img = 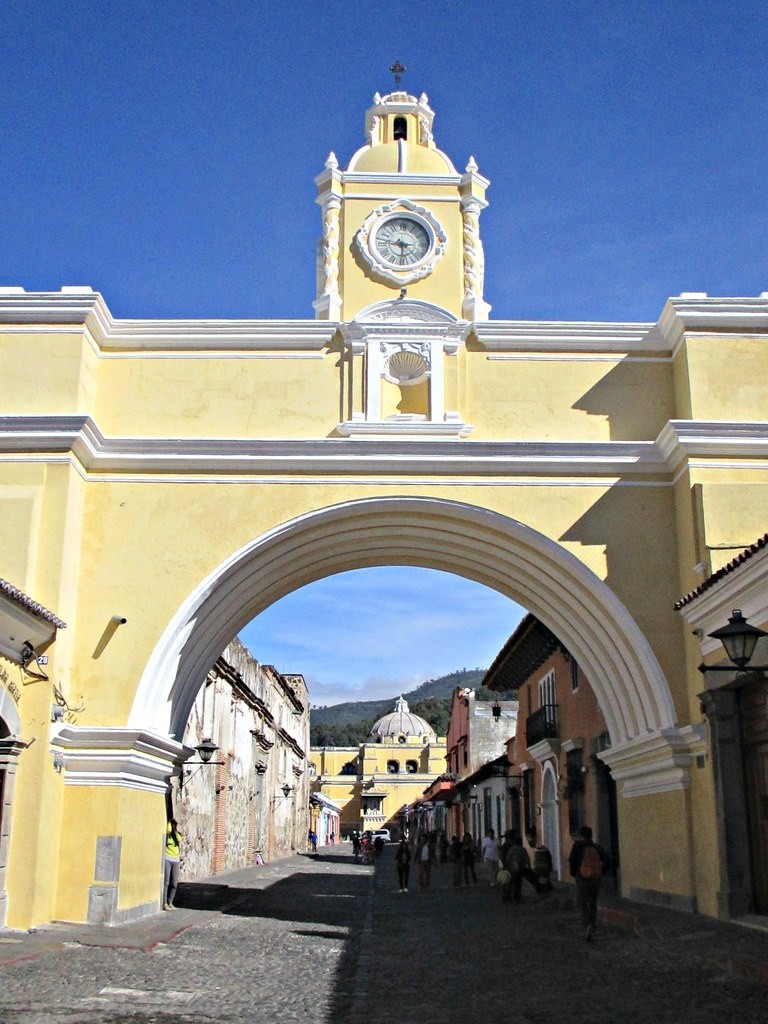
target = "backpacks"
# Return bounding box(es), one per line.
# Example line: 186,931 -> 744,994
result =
579,840 -> 603,879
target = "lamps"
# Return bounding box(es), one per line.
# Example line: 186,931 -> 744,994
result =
273,784 -> 295,812
178,739 -> 225,790
698,608 -> 768,708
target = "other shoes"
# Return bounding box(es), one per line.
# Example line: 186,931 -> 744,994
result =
403,888 -> 409,893
398,889 -> 402,893
584,923 -> 594,942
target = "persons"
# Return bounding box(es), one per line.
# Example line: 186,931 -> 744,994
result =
394,839 -> 410,894
499,827 -> 547,905
480,829 -> 499,888
568,826 -> 616,940
310,831 -> 318,852
413,833 -> 434,890
330,831 -> 335,846
163,818 -> 184,911
450,835 -> 461,888
352,830 -> 385,864
461,833 -> 476,886
438,829 -> 449,868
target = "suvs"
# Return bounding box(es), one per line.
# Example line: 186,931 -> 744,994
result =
359,829 -> 390,843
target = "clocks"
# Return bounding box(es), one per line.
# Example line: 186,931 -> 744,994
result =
369,212 -> 436,270
